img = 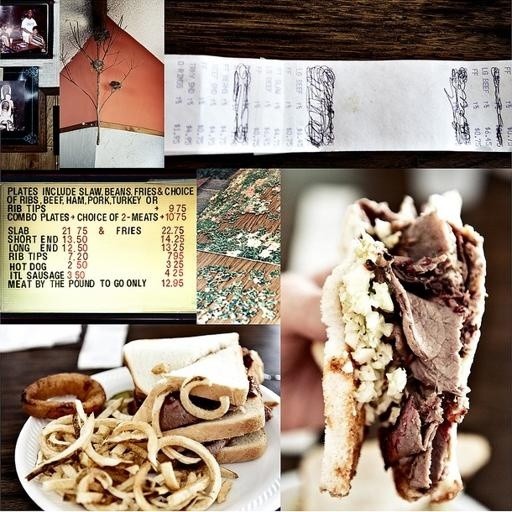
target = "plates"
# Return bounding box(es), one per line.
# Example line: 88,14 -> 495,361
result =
15,363 -> 281,509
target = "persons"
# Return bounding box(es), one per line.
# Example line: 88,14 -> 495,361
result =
29,25 -> 46,49
0,101 -> 15,132
0,85 -> 15,116
21,10 -> 40,45
281,262 -> 340,435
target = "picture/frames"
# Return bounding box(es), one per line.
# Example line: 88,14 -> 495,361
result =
0,0 -> 56,155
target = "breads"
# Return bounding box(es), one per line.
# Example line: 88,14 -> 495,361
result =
124,332 -> 267,464
